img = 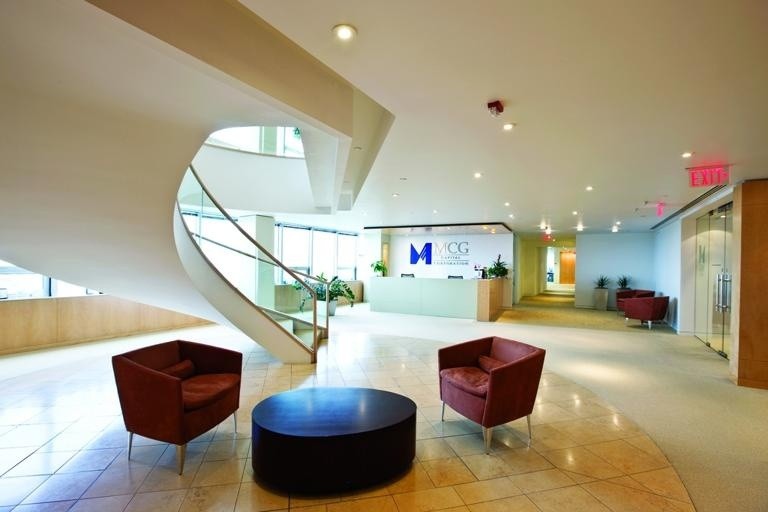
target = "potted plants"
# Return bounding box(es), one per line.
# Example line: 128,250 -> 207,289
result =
292,274 -> 356,316
616,275 -> 634,311
370,260 -> 387,277
486,253 -> 509,280
593,275 -> 610,311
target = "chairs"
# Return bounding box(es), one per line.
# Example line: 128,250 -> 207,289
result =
112,340 -> 243,475
623,297 -> 669,330
438,335 -> 546,455
616,289 -> 655,315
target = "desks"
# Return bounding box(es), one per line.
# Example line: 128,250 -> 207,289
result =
251,386 -> 416,499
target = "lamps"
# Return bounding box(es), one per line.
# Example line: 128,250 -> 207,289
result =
690,166 -> 731,187
